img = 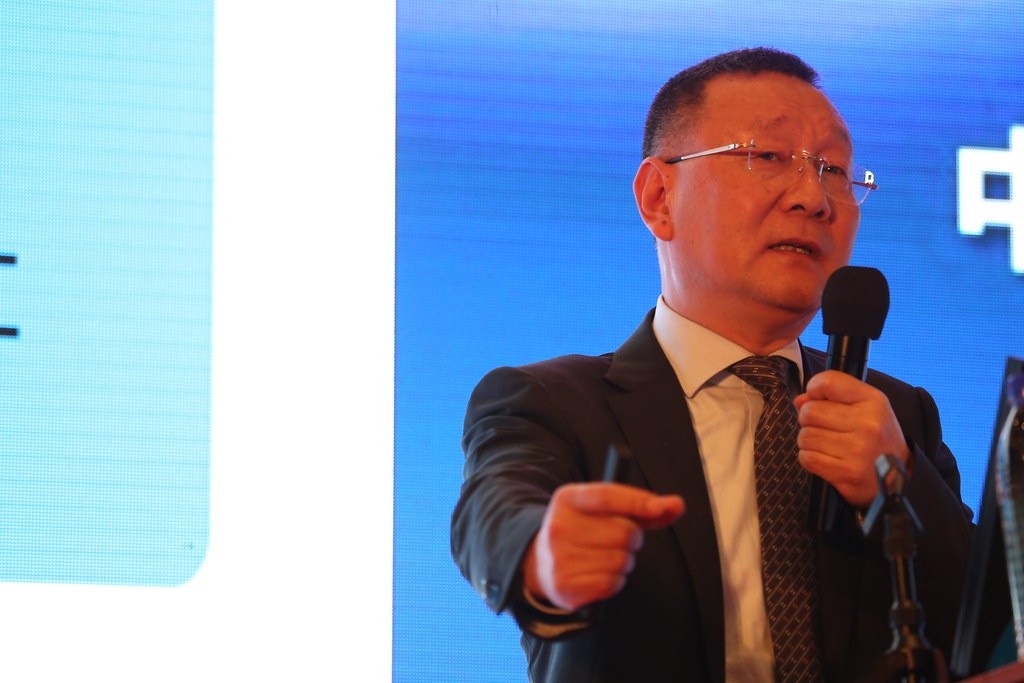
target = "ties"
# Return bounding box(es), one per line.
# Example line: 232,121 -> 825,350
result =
727,357 -> 824,683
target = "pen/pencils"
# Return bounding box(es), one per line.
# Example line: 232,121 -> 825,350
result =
601,444 -> 619,482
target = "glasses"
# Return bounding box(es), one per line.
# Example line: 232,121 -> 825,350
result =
665,139 -> 877,204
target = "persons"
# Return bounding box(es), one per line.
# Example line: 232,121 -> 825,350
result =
449,46 -> 1012,683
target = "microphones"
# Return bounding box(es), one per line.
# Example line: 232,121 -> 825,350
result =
807,265 -> 891,539
